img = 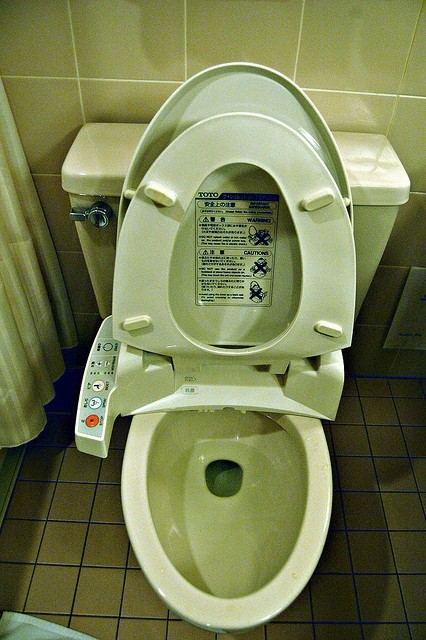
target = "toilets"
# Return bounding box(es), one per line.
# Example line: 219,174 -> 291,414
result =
61,60 -> 412,635
